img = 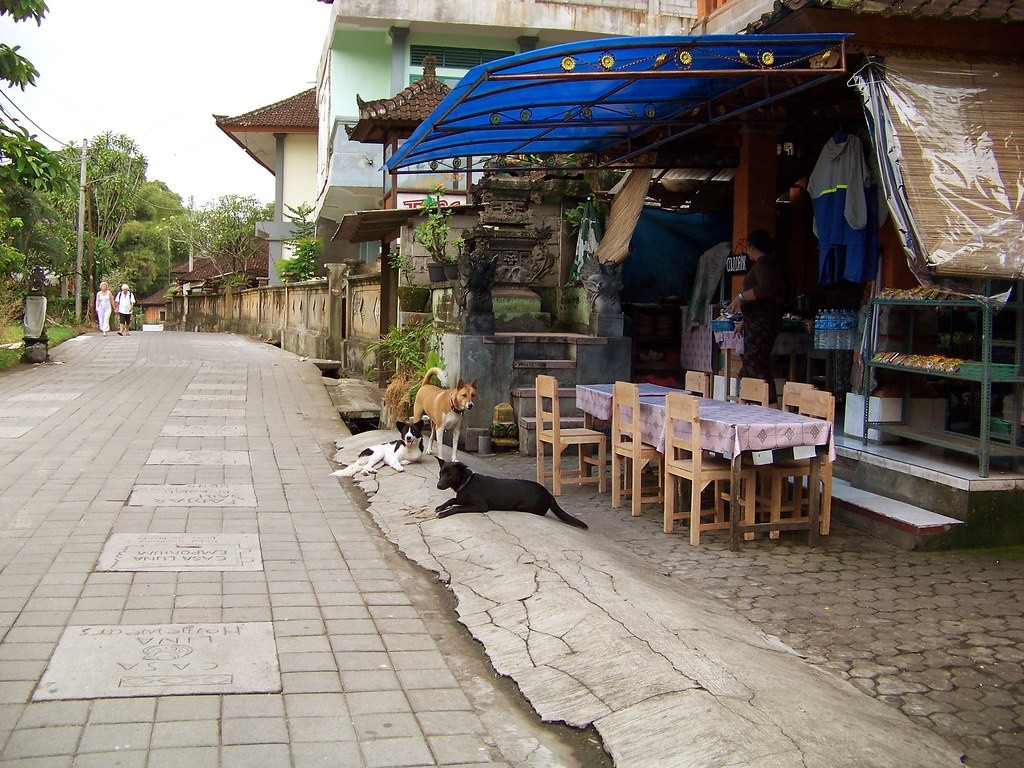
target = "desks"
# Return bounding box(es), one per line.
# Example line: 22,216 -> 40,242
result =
576,383 -> 831,552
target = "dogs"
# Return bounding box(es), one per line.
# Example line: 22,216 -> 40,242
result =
412,367 -> 479,462
434,455 -> 589,530
329,419 -> 424,478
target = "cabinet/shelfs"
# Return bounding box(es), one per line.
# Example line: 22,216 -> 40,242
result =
863,297 -> 1024,478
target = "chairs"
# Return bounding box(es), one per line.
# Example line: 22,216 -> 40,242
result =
612,371 -> 835,546
536,375 -> 606,496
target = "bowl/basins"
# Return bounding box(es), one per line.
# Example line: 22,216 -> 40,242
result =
710,320 -> 735,332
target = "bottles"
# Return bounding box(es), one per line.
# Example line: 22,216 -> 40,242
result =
814,309 -> 858,350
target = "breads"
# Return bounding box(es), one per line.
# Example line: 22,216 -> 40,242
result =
872,351 -> 968,372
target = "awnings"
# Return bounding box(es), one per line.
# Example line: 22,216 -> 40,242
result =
377,30 -> 856,176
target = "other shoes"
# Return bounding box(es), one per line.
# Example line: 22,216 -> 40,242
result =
117,332 -> 124,336
126,333 -> 130,335
103,332 -> 107,337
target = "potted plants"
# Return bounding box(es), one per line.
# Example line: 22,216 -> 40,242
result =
388,181 -> 466,312
228,273 -> 252,290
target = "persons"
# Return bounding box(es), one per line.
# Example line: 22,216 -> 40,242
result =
95,282 -> 117,336
115,284 -> 136,336
723,230 -> 783,413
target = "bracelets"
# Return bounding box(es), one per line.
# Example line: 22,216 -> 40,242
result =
114,309 -> 116,310
737,292 -> 745,303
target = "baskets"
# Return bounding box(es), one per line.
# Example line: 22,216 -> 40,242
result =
991,418 -> 1014,436
782,318 -> 802,332
959,361 -> 1021,380
711,320 -> 733,330
